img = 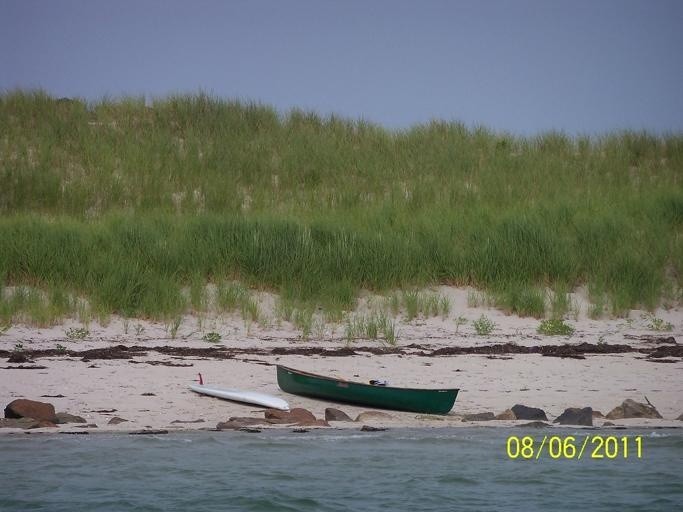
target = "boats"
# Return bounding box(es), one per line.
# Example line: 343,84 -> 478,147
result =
275,363 -> 461,417
